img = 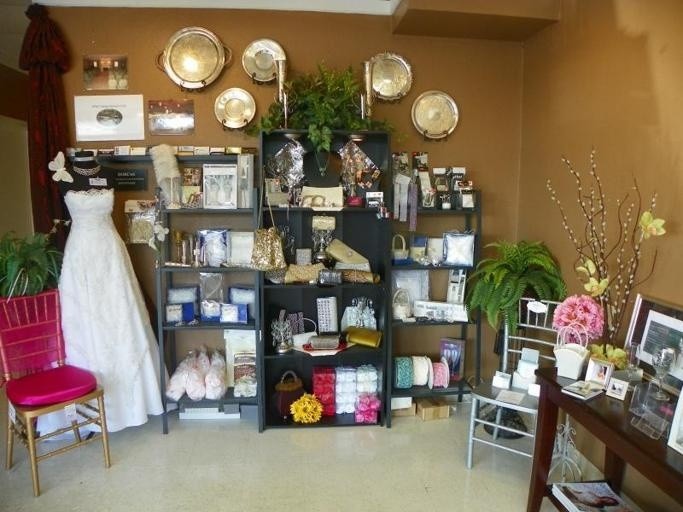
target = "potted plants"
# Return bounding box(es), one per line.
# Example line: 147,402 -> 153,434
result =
463,234 -> 568,375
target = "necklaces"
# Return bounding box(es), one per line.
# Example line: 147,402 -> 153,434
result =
72,165 -> 100,176
314,150 -> 331,177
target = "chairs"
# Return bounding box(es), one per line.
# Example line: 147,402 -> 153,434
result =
464,297 -> 565,470
0,286 -> 113,498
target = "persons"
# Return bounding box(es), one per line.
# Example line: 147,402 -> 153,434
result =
555,484 -> 618,512
37,151 -> 178,441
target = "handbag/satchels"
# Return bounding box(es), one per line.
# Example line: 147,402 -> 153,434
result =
553,321 -> 589,381
325,238 -> 368,265
393,237 -> 410,265
343,325 -> 382,348
270,373 -> 308,418
298,185 -> 344,207
284,263 -> 328,285
288,317 -> 318,350
249,165 -> 288,271
392,288 -> 412,322
309,336 -> 341,350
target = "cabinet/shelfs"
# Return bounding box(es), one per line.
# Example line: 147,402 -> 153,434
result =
154,185 -> 264,436
386,188 -> 483,429
259,128 -> 386,434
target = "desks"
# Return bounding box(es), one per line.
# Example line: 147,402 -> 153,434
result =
526,361 -> 682,512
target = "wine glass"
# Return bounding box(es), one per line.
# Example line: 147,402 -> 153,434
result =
651,346 -> 676,400
622,341 -> 644,394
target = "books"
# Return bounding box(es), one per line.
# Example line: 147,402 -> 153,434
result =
551,481 -> 632,512
561,380 -> 603,400
